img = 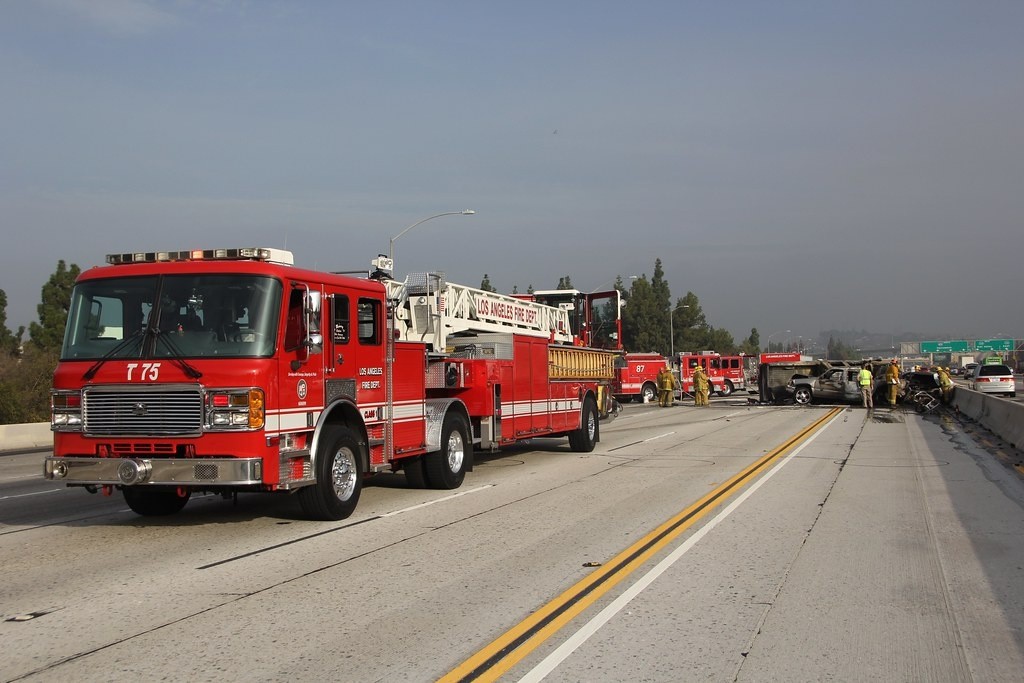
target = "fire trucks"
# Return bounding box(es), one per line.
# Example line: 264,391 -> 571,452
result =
611,349 -> 756,403
757,352 -> 818,404
43,247 -> 618,522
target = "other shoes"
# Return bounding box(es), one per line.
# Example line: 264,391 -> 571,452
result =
703,404 -> 711,406
694,404 -> 701,407
868,406 -> 873,409
660,406 -> 665,407
860,406 -> 867,408
665,405 -> 673,408
889,404 -> 897,409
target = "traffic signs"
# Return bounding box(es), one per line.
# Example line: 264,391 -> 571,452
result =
919,339 -> 968,354
973,338 -> 1015,352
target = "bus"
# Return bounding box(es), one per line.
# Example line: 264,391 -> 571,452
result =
982,356 -> 1003,365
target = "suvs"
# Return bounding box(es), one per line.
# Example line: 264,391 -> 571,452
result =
784,365 -> 908,406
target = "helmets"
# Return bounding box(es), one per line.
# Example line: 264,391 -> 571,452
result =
936,367 -> 943,371
945,367 -> 950,371
890,359 -> 896,363
659,367 -> 664,371
693,367 -> 699,371
893,358 -> 898,362
666,366 -> 671,371
698,366 -> 703,370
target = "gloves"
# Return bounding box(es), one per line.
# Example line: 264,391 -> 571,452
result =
674,387 -> 676,390
897,380 -> 900,384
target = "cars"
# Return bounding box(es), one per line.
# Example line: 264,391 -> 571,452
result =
963,363 -> 979,379
949,363 -> 959,376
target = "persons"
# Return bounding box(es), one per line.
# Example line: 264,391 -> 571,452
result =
657,366 -> 679,407
885,359 -> 901,408
915,366 -> 951,405
857,365 -> 873,408
693,366 -> 712,407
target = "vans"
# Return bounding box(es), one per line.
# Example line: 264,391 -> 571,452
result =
967,364 -> 1016,397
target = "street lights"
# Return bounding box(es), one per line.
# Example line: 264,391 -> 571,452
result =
670,305 -> 690,356
767,330 -> 792,353
389,209 -> 476,259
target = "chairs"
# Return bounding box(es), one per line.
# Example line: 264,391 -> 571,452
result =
181,313 -> 203,331
229,301 -> 256,326
